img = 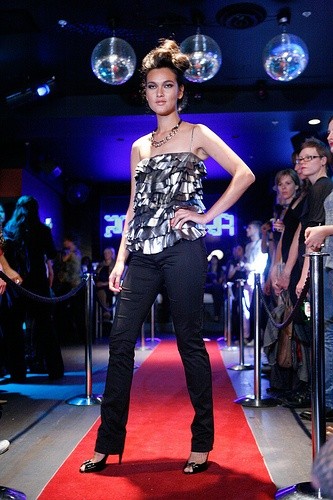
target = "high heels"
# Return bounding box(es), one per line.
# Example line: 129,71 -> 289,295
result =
183,451 -> 209,474
79,450 -> 122,472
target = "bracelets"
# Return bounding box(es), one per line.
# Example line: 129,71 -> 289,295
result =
268,238 -> 273,241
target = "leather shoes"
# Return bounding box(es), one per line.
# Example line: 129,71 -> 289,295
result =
300,407 -> 333,422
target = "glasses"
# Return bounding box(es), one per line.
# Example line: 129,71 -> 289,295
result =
296,155 -> 322,163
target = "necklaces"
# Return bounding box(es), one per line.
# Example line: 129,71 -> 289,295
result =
148,118 -> 183,147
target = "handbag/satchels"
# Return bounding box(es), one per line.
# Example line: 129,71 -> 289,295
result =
277,289 -> 295,368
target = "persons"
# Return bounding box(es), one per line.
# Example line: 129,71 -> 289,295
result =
78,38 -> 256,473
0,116 -> 333,500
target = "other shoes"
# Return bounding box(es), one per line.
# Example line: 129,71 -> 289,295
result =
0,439 -> 11,454
263,359 -> 311,406
